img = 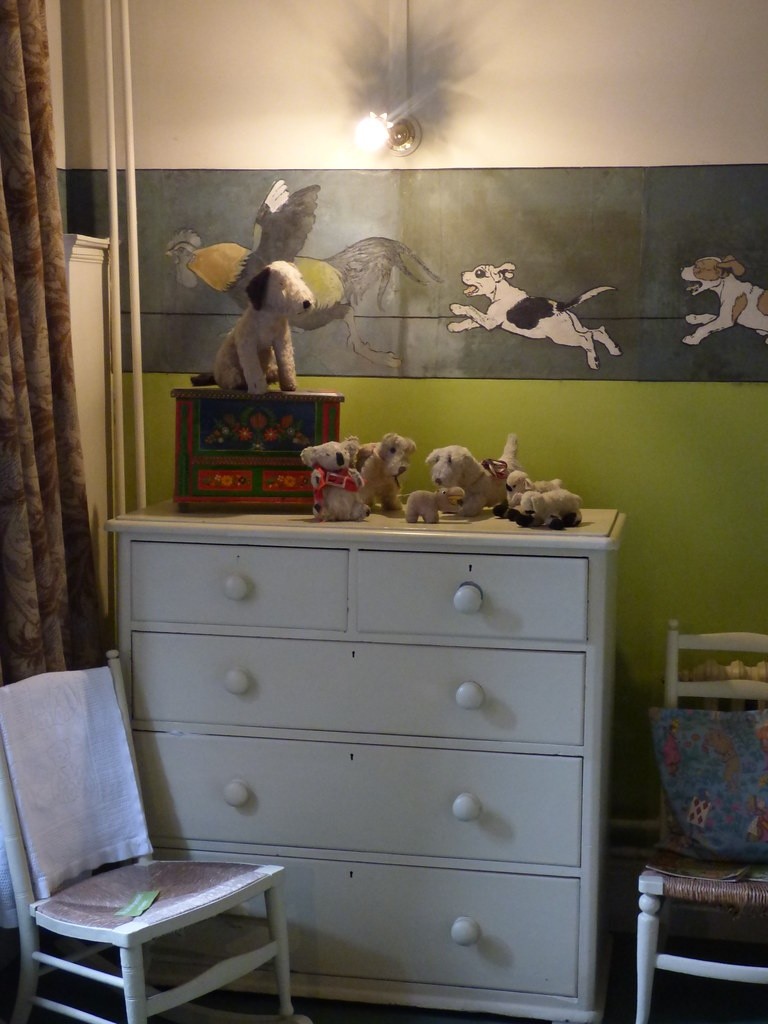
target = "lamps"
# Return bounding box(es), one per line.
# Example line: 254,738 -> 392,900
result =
356,112 -> 422,156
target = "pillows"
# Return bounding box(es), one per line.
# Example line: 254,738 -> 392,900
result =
651,707 -> 768,858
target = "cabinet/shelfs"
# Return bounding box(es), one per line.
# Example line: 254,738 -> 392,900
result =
103,500 -> 628,1024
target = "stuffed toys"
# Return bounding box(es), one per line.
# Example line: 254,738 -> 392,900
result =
302,437 -> 371,520
407,487 -> 464,523
357,433 -> 416,510
494,471 -> 562,520
515,490 -> 583,529
190,261 -> 315,393
425,434 -> 520,516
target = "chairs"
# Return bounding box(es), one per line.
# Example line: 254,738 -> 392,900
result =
635,619 -> 768,1024
0,648 -> 313,1024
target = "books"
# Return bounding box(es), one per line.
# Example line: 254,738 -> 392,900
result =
646,852 -> 768,883
